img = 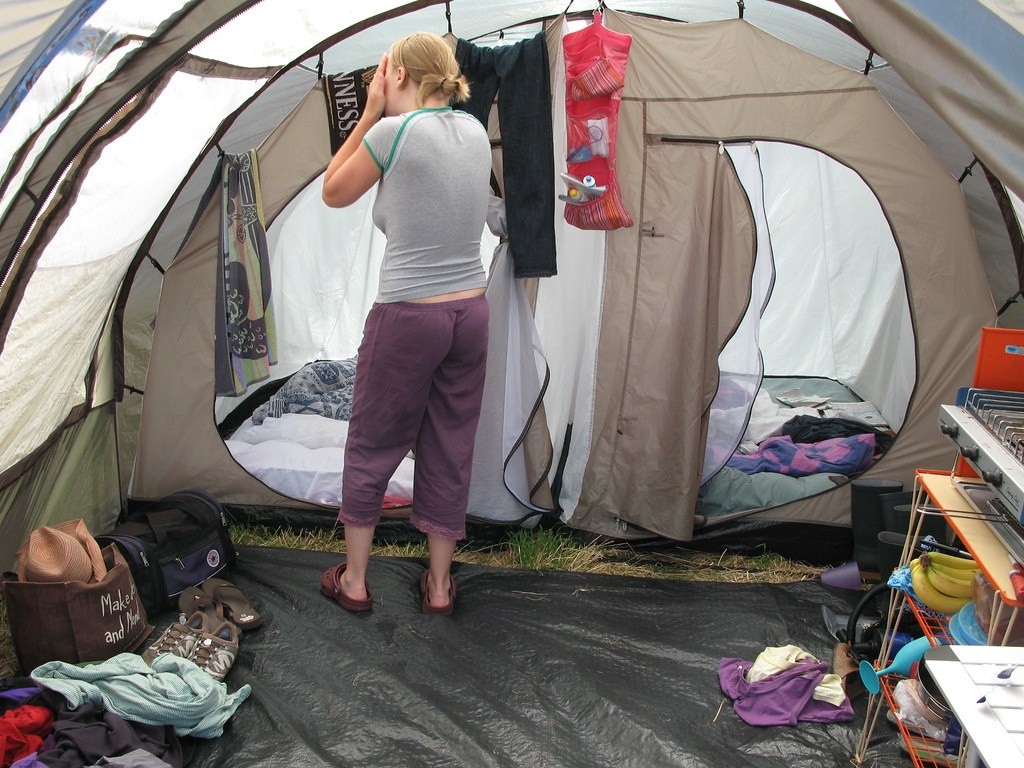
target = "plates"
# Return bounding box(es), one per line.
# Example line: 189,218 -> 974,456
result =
949,611 -> 971,645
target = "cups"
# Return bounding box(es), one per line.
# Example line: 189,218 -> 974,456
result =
822,561 -> 862,590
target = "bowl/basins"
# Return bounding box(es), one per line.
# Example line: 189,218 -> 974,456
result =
959,601 -> 987,646
911,688 -> 948,730
917,656 -> 953,720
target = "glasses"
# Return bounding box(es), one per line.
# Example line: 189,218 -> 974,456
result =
565,124 -> 602,161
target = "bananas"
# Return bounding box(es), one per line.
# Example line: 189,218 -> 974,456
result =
909,551 -> 984,616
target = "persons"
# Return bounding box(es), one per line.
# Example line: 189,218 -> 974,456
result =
321,32 -> 492,616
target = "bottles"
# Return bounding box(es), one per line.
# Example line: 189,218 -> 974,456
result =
854,629 -> 916,659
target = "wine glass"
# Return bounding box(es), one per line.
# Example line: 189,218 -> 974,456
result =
859,636 -> 942,694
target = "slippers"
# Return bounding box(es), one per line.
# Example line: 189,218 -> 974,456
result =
201,578 -> 262,629
421,569 -> 455,614
178,585 -> 243,642
321,562 -> 373,611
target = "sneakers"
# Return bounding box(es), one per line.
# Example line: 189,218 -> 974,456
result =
142,611 -> 210,669
186,621 -> 238,681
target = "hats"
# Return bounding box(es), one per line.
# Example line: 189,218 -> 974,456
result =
16,518 -> 107,584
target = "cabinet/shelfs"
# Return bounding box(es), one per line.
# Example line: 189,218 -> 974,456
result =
849,470 -> 1024,768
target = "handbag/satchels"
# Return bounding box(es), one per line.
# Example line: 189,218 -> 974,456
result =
92,488 -> 237,621
1,543 -> 155,677
